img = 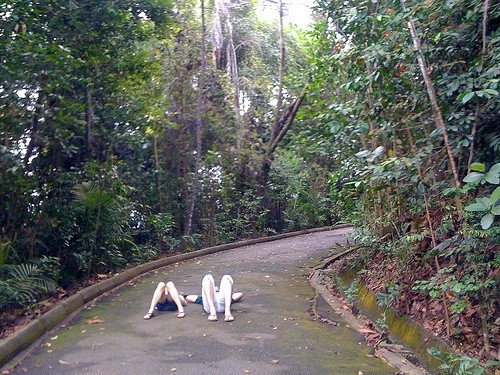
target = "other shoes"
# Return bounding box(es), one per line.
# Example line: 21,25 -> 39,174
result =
176,310 -> 186,318
207,314 -> 218,321
143,311 -> 154,319
224,314 -> 235,322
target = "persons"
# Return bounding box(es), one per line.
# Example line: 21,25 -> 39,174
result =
186,274 -> 244,321
143,280 -> 187,317
